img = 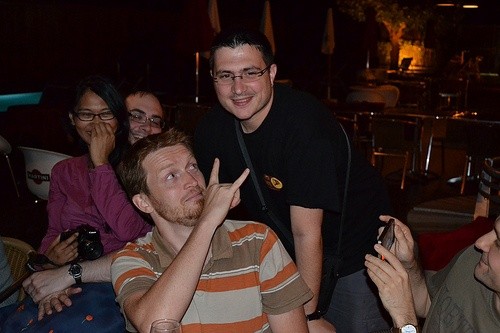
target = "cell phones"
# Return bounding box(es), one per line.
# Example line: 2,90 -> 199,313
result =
377,218 -> 396,261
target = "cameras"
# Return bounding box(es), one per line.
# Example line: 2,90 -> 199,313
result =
60,224 -> 103,264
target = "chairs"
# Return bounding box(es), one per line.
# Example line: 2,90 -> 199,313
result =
0,236 -> 37,303
18,146 -> 76,202
356,112 -> 420,190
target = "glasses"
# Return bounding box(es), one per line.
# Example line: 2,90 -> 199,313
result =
73,111 -> 119,121
212,66 -> 270,84
128,111 -> 165,128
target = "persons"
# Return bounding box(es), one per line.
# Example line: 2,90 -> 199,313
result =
359,202 -> 500,332
189,46 -> 393,333
28,77 -> 151,275
107,125 -> 313,332
21,72 -> 173,320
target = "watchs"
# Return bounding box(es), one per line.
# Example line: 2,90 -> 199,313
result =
390,321 -> 420,333
67,261 -> 85,286
